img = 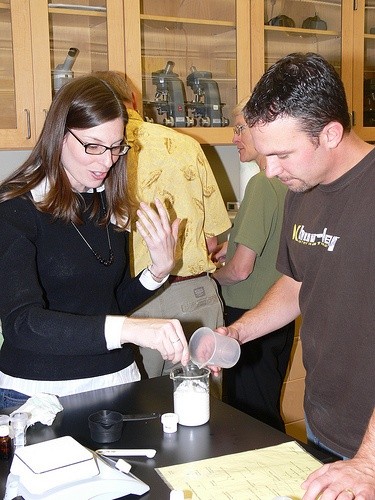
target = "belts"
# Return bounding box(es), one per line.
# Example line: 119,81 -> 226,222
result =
168,272 -> 207,284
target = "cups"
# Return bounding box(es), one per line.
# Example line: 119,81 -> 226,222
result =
188,326 -> 241,369
169,364 -> 211,427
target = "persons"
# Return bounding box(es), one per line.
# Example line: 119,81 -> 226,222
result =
197,53 -> 375,500
212,107 -> 295,434
0,74 -> 188,408
94,69 -> 231,401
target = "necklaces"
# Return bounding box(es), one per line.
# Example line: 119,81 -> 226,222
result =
69,193 -> 114,266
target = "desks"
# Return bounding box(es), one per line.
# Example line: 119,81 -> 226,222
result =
0,375 -> 341,500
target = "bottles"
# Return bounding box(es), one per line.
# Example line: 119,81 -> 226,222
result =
161,412 -> 179,433
0,413 -> 28,471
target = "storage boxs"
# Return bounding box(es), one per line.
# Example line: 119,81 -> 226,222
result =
9,435 -> 100,494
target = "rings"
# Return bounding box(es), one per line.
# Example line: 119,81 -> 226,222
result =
344,487 -> 355,498
170,338 -> 181,343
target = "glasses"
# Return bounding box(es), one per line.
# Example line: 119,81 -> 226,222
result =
64,127 -> 130,156
232,123 -> 248,135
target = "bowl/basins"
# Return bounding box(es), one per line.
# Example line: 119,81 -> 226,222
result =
88,410 -> 124,443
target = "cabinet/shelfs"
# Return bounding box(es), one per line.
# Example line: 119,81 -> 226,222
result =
0,0 -> 125,150
353,0 -> 375,142
123,0 -> 355,145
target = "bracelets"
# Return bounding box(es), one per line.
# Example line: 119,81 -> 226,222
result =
147,265 -> 167,281
